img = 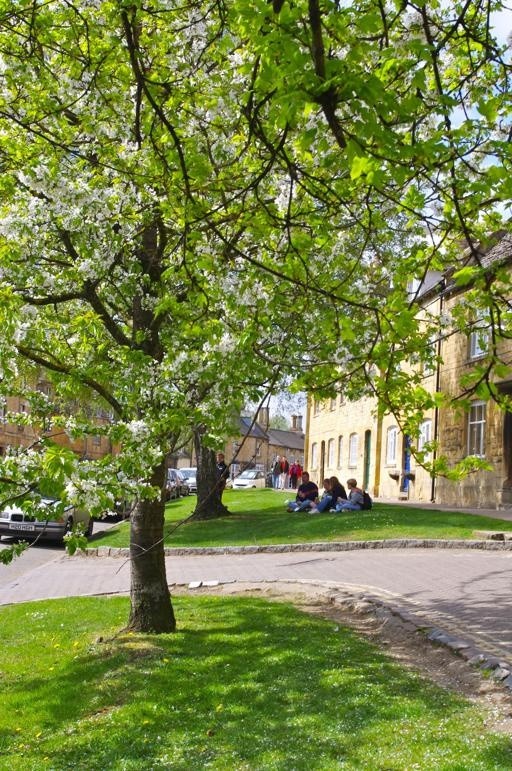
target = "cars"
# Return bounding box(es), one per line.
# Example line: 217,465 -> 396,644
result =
0,478 -> 97,546
74,463 -> 198,520
232,468 -> 266,489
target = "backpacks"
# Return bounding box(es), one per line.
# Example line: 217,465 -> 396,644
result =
360,490 -> 372,510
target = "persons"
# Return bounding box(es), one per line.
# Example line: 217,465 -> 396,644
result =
310,478 -> 332,507
306,476 -> 348,516
215,453 -> 231,503
269,456 -> 304,492
328,479 -> 364,515
286,472 -> 319,513
